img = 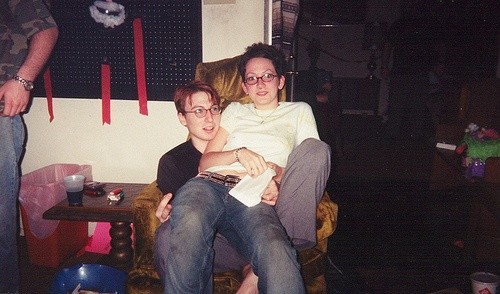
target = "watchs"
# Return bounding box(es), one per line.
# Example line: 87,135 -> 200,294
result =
14,75 -> 34,91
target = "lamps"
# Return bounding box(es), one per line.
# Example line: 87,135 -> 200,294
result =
361,22 -> 389,80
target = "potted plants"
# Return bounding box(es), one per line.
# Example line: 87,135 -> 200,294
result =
470,272 -> 499,294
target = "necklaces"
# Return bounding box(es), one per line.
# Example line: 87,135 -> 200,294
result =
254,107 -> 277,126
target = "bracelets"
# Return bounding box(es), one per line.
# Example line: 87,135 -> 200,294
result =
235,147 -> 248,162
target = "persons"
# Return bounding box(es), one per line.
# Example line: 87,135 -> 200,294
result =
154,81 -> 331,294
164,43 -> 322,294
0,0 -> 58,294
382,0 -> 447,142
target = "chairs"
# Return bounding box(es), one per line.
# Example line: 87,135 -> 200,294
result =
126,48 -> 338,294
429,79 -> 500,285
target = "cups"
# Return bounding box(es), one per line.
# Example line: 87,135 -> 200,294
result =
64,175 -> 86,205
469,272 -> 499,294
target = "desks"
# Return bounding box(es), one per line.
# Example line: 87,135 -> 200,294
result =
42,183 -> 149,273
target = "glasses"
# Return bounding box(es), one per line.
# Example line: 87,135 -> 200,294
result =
185,105 -> 222,117
244,73 -> 279,86
